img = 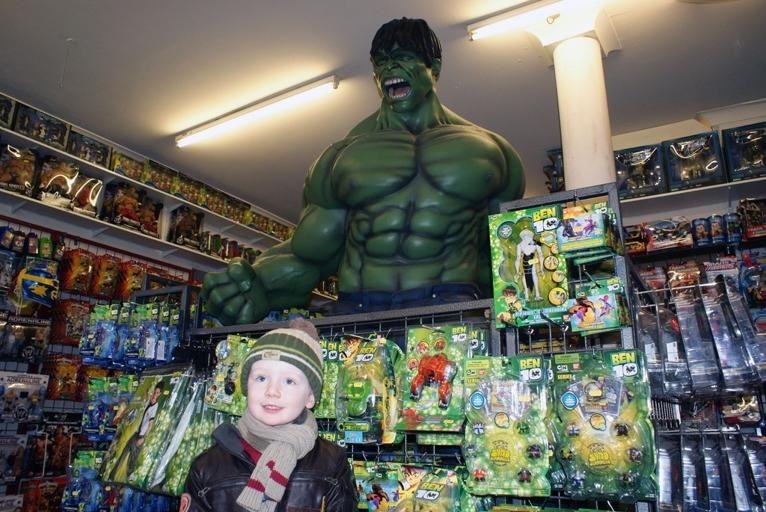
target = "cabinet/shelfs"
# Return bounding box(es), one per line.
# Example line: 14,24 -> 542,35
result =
0,122 -> 766,512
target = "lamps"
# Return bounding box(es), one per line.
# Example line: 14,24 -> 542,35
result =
174,74 -> 338,149
466,0 -> 594,44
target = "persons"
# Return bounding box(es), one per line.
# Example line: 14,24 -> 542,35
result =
177,313 -> 355,512
198,15 -> 526,326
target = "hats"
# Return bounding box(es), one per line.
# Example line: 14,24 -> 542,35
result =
240,314 -> 325,408
